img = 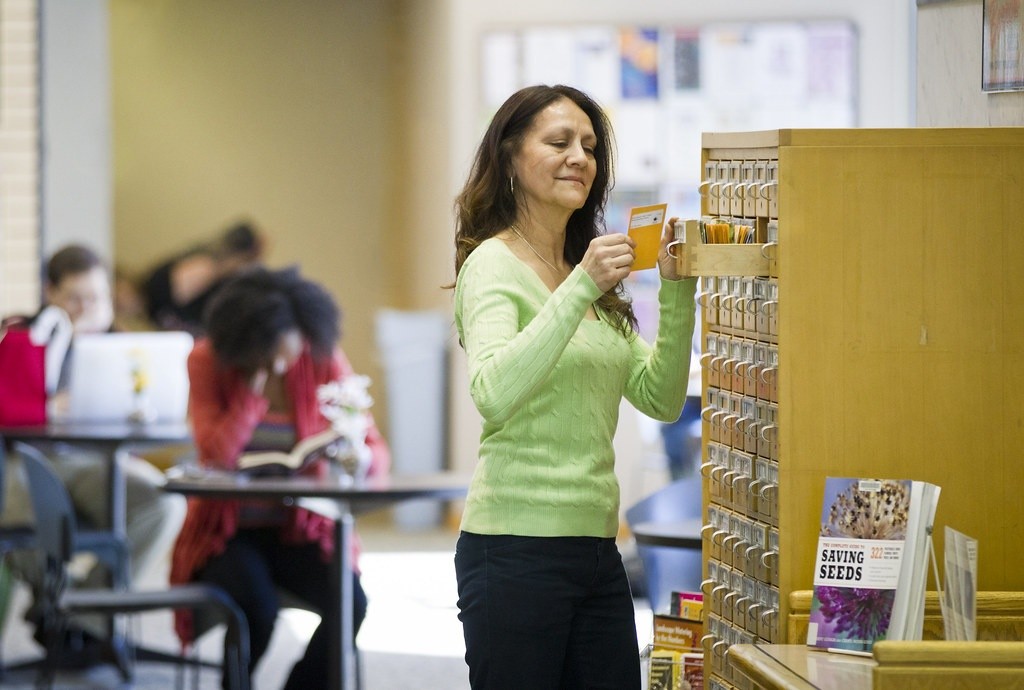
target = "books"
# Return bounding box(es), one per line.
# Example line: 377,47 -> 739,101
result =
234,426 -> 346,474
646,589 -> 705,690
806,476 -> 941,654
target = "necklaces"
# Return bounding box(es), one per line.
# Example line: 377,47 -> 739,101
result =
511,225 -> 568,280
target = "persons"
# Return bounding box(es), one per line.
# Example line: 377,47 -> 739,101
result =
439,83 -> 698,690
1,243 -> 188,639
140,219 -> 265,326
167,267 -> 389,690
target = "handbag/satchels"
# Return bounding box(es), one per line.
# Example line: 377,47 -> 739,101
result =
2,306 -> 74,432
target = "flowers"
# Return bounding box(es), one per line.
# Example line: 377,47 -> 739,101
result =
314,375 -> 375,415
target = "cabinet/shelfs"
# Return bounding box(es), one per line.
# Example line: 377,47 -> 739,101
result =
668,129 -> 1024,690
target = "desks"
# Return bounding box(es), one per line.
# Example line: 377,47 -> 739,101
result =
726,642 -> 873,690
0,428 -> 196,641
161,464 -> 468,690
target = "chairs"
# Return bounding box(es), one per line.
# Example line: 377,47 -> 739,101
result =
0,442 -> 251,690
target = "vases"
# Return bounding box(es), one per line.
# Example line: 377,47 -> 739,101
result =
329,413 -> 372,485
